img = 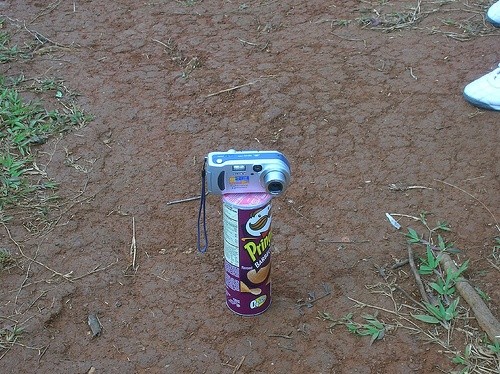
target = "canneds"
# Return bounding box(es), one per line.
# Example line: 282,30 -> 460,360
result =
223,192 -> 273,316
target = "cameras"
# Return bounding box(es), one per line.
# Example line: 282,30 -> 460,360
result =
207,149 -> 290,198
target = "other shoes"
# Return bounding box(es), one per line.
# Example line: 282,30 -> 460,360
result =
486,0 -> 500,25
459,64 -> 500,111
